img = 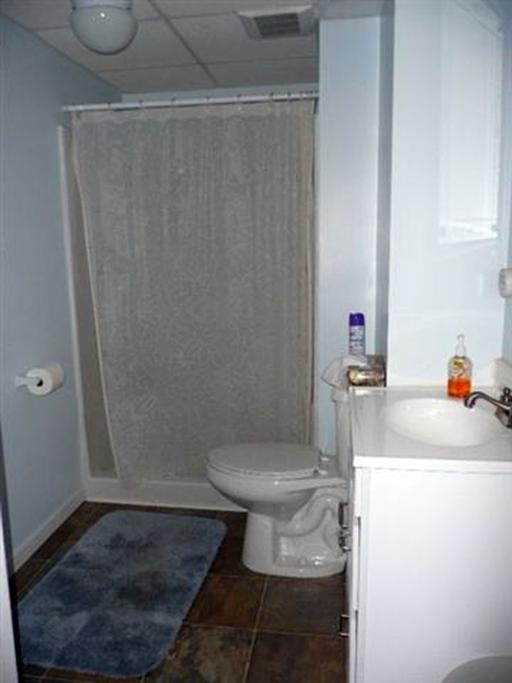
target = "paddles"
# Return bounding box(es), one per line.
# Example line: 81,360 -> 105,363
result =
17,511 -> 227,679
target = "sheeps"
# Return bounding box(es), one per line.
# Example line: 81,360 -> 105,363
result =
464,391 -> 511,415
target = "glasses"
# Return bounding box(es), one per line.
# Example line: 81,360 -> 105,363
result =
71,0 -> 138,54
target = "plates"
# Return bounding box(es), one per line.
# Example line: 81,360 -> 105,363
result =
338,396 -> 512,683
